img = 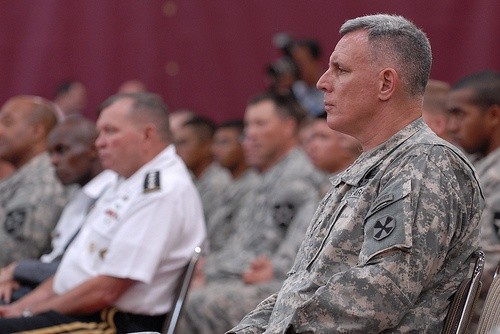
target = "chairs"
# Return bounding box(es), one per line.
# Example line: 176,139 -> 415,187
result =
441,250 -> 500,334
164,245 -> 201,334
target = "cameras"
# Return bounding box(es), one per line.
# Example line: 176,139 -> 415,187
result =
266,33 -> 297,77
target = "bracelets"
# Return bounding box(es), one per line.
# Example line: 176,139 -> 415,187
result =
22,306 -> 33,318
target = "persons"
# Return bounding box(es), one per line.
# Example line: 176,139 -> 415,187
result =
225,14 -> 486,334
0,80 -> 147,306
422,71 -> 500,334
0,93 -> 208,334
170,40 -> 363,334
